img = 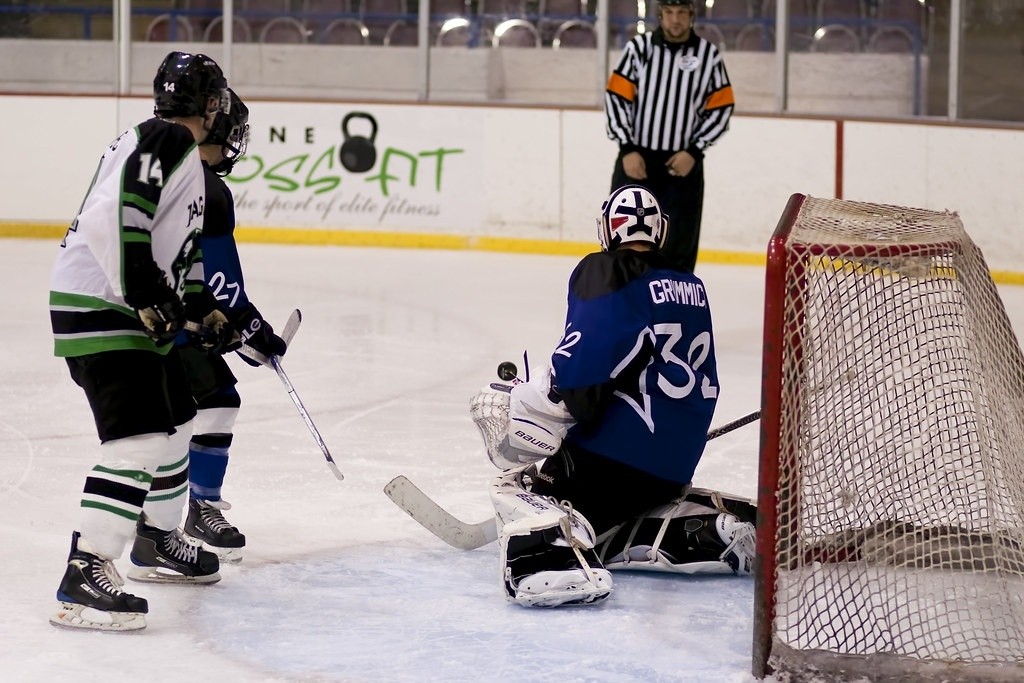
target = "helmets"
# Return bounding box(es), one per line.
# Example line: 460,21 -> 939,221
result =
659,0 -> 693,6
197,87 -> 249,171
596,183 -> 671,252
153,50 -> 228,112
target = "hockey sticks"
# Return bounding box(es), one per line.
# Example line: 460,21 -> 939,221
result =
269,354 -> 345,482
179,308 -> 304,372
381,369 -> 859,555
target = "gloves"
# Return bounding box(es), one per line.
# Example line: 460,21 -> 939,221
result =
226,301 -> 287,367
183,290 -> 233,354
123,267 -> 188,347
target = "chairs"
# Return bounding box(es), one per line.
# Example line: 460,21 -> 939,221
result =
145,0 -> 934,52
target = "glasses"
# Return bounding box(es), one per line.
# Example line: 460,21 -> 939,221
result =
216,90 -> 231,114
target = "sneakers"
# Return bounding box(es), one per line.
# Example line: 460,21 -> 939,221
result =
682,512 -> 756,577
181,496 -> 246,560
48,530 -> 149,631
514,560 -> 614,608
126,511 -> 222,586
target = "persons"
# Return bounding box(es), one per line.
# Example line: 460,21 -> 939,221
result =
604,0 -> 735,273
48,52 -> 286,631
470,184 -> 756,608
